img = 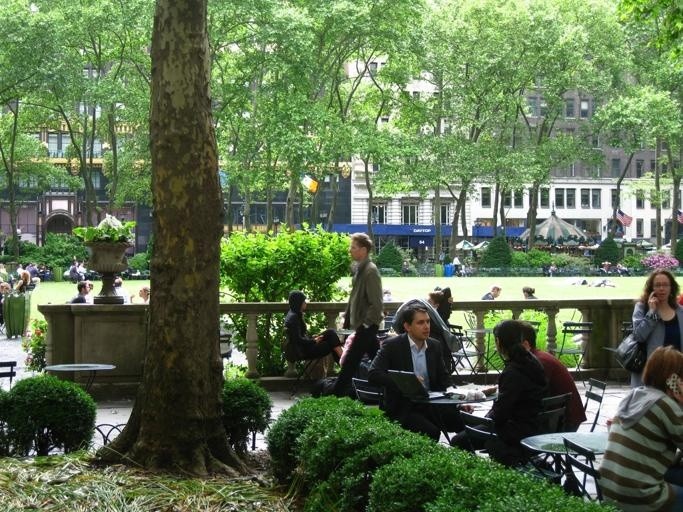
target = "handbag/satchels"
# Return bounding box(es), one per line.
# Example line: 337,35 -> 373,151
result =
613,332 -> 647,374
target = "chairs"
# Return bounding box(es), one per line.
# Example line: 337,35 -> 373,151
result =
460,410 -> 559,482
549,322 -> 593,388
584,377 -> 607,433
0,360 -> 16,389
448,324 -> 485,383
283,324 -> 323,395
377,328 -> 389,341
352,377 -> 389,418
532,322 -> 541,335
563,435 -> 602,504
533,392 -> 572,434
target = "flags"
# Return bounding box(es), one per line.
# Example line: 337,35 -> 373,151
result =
616,207 -> 633,228
672,209 -> 682,225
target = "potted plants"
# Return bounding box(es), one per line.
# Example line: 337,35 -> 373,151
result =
72,214 -> 135,303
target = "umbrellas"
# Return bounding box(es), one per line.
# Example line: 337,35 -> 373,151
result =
471,240 -> 490,249
454,239 -> 475,250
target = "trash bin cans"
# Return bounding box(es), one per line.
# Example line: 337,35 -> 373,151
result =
4,294 -> 29,339
435,264 -> 453,277
53,267 -> 63,282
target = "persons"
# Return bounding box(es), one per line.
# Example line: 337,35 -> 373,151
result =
129,287 -> 150,305
548,261 -> 557,278
283,291 -> 342,364
588,279 -> 614,288
425,285 -> 452,325
613,263 -> 631,277
328,230 -> 389,399
600,260 -> 612,276
113,276 -> 122,296
543,263 -> 549,277
571,279 -> 590,287
593,343 -> 682,511
451,255 -> 461,276
0,256 -> 94,327
0,231 -> 6,257
517,321 -> 587,432
400,261 -> 412,276
447,319 -> 548,458
438,246 -> 447,265
460,261 -> 472,277
521,287 -> 537,300
366,301 -> 474,443
627,267 -> 682,388
16,226 -> 22,241
481,285 -> 502,300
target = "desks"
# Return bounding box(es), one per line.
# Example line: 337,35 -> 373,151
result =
46,363 -> 116,394
